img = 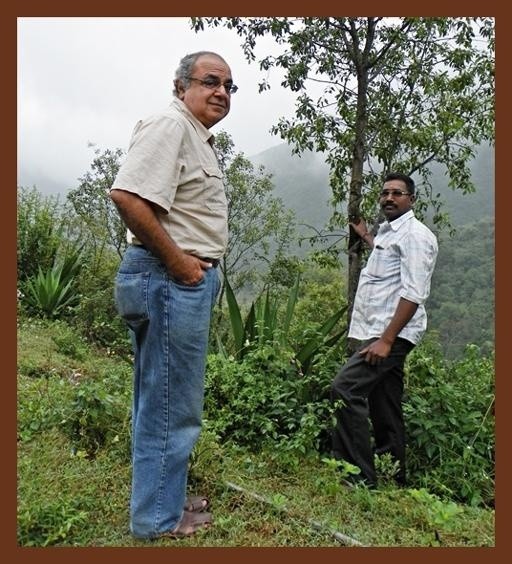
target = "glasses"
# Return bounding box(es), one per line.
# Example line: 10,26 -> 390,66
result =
381,190 -> 412,197
184,76 -> 238,94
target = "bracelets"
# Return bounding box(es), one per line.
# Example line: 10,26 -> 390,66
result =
363,232 -> 371,237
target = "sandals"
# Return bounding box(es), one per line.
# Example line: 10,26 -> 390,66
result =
173,495 -> 210,536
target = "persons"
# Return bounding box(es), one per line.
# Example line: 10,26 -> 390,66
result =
110,51 -> 238,539
330,173 -> 439,487
